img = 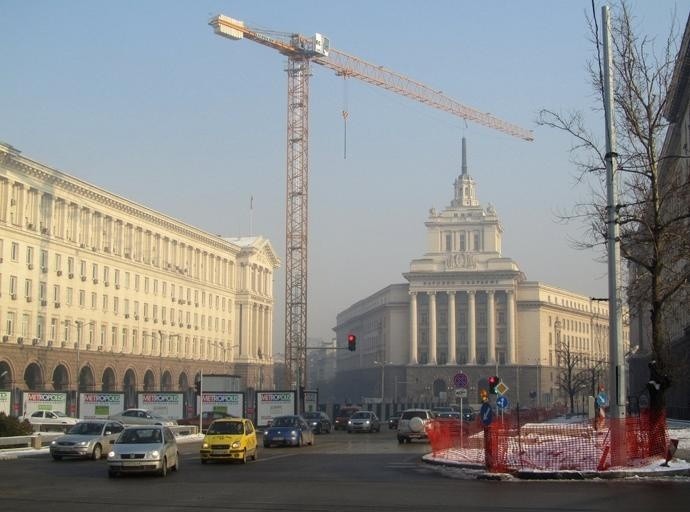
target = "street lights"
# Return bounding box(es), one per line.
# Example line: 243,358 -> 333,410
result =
211,341 -> 240,374
61,319 -> 94,384
143,330 -> 179,393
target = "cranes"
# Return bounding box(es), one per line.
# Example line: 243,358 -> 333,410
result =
208,13 -> 534,392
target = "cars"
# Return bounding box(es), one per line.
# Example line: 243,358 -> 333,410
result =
263,408 -> 380,448
200,418 -> 258,465
19,410 -> 80,426
108,408 -> 179,426
388,406 -> 476,444
50,420 -> 125,461
107,425 -> 179,479
177,411 -> 239,429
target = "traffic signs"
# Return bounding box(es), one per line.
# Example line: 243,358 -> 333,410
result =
456,389 -> 467,398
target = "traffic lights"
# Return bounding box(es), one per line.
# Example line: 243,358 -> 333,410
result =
348,335 -> 355,351
488,376 -> 498,394
